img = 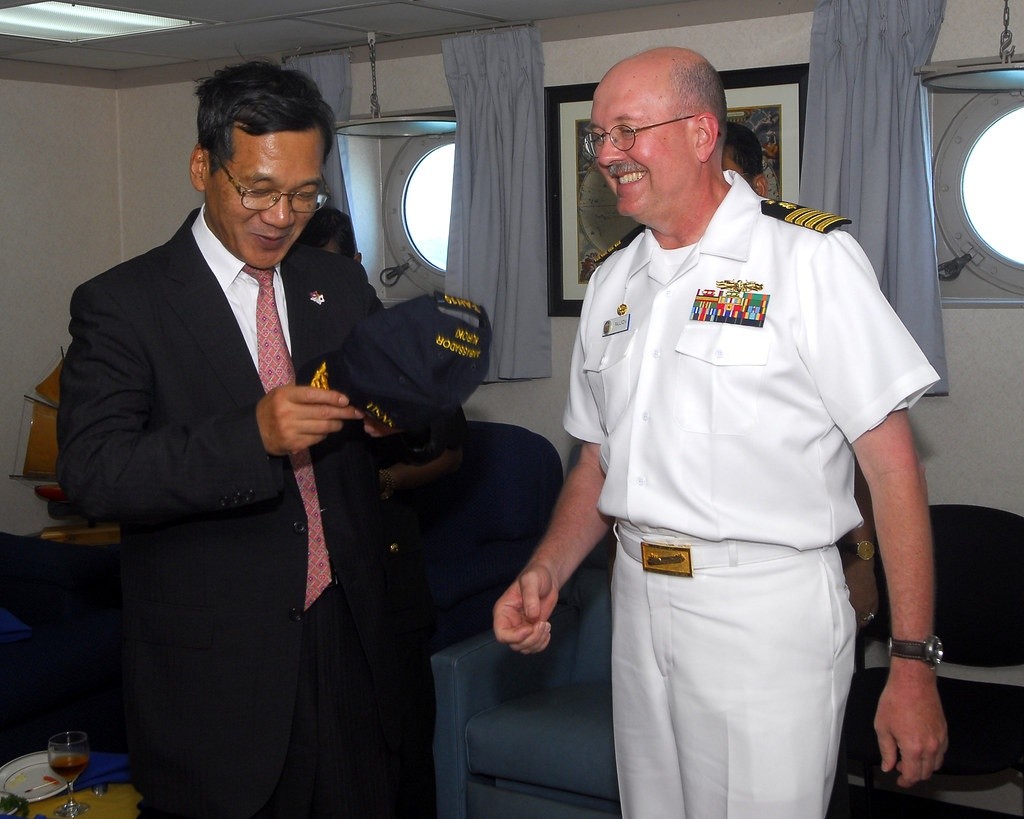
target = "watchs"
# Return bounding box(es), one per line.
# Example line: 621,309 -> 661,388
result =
839,540 -> 878,560
887,635 -> 945,670
379,468 -> 395,500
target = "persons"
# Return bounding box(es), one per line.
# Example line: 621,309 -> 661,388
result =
490,46 -> 949,819
58,62 -> 421,819
719,121 -> 879,636
295,208 -> 469,694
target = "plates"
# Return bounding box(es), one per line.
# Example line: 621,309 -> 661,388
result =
0,791 -> 21,816
0,750 -> 78,803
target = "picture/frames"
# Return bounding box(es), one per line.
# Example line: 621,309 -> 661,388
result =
546,61 -> 810,318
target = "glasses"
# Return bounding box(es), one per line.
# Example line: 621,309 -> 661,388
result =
220,163 -> 332,213
584,115 -> 696,159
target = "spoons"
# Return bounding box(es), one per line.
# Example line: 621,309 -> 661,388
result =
92,783 -> 108,800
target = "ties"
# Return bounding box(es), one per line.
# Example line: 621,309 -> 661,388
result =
242,265 -> 331,611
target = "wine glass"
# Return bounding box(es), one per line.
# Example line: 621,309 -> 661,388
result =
47,731 -> 89,818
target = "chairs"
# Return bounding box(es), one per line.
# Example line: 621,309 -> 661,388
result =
834,505 -> 1024,819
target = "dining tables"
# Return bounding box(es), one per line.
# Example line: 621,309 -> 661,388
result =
0,752 -> 144,819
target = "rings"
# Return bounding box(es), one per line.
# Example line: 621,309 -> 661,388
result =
863,612 -> 874,622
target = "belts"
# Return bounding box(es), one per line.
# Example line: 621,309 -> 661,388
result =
616,526 -> 803,578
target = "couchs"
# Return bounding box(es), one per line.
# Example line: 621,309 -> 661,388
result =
428,443 -> 622,819
0,533 -> 127,755
419,418 -> 562,651
